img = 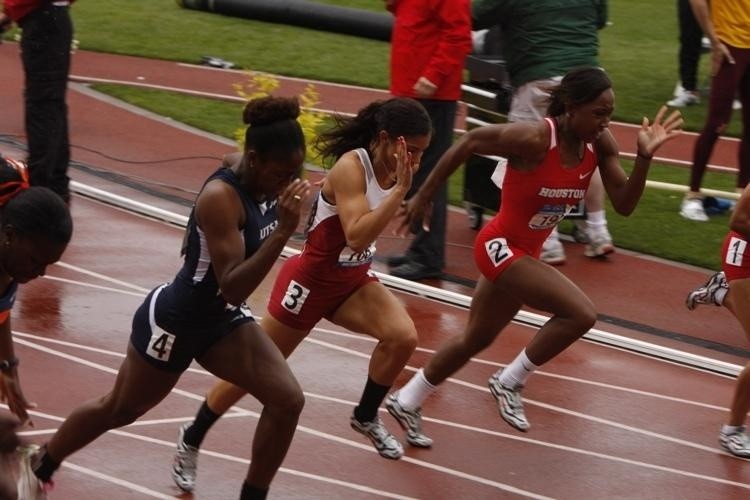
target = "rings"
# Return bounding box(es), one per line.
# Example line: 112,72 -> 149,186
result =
292,194 -> 302,201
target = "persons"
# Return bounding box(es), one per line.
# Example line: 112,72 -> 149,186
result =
385,1 -> 615,263
168,95 -> 437,493
13,91 -> 313,499
0,0 -> 80,206
0,155 -> 78,451
686,180 -> 750,458
383,65 -> 687,452
666,0 -> 750,221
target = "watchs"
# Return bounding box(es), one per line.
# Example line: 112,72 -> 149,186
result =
0,357 -> 20,372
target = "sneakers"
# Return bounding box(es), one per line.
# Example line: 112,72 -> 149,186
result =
387,239 -> 425,266
487,367 -> 531,433
389,257 -> 444,281
540,238 -> 566,266
673,83 -> 687,97
171,423 -> 200,492
677,193 -> 710,221
716,426 -> 750,458
667,91 -> 700,109
686,270 -> 729,311
349,405 -> 406,460
15,443 -> 54,500
383,388 -> 434,448
583,232 -> 614,256
